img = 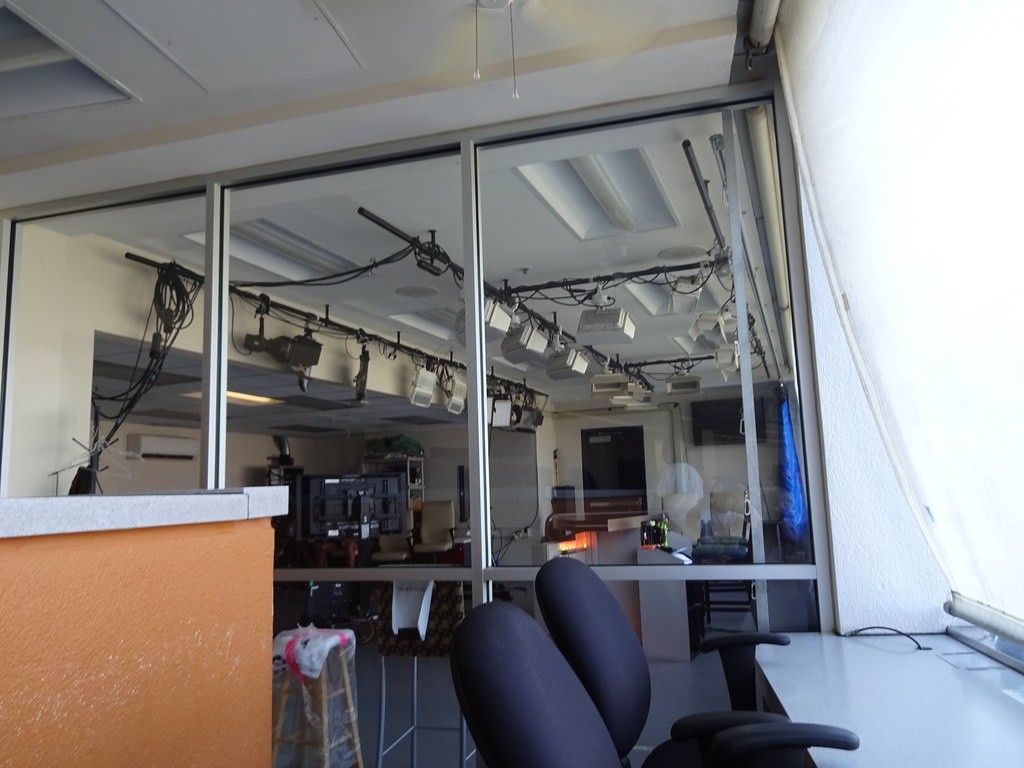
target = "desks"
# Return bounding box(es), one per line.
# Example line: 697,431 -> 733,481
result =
756,632 -> 1023,768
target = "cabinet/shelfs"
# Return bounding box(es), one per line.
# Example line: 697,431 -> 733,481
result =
361,456 -> 426,510
301,472 -> 408,539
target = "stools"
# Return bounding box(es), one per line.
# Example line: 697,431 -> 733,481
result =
273,628 -> 364,768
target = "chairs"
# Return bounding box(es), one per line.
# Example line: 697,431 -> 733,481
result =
450,601 -> 860,768
412,500 -> 457,564
371,531 -> 413,567
692,491 -> 758,638
536,557 -> 791,768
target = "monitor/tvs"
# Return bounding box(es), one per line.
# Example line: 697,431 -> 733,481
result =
296,473 -> 404,568
692,396 -> 767,447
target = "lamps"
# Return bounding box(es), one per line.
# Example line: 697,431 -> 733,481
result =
409,369 -> 436,407
446,380 -> 467,415
457,272 -> 740,403
